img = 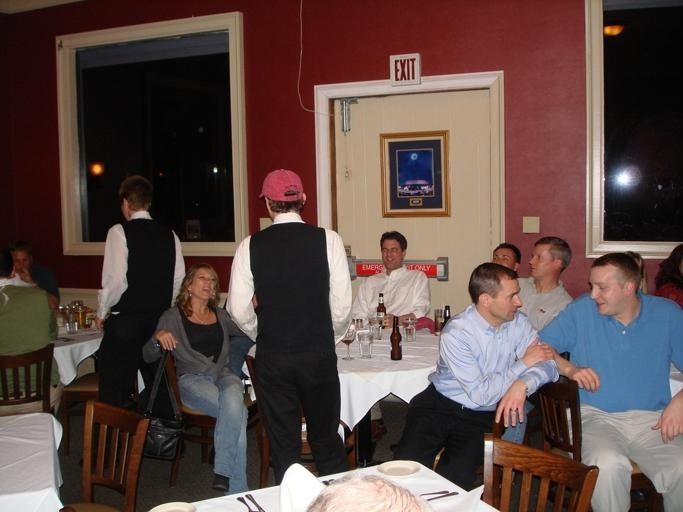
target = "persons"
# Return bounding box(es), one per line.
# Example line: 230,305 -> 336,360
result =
224,166 -> 354,486
651,242 -> 683,309
623,250 -> 648,294
94,175 -> 187,439
351,230 -> 432,328
537,252 -> 683,512
514,236 -> 574,331
492,242 -> 521,273
391,258 -> 560,491
0,244 -> 59,401
142,261 -> 255,495
10,239 -> 60,310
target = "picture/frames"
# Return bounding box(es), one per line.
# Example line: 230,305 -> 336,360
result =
379,129 -> 451,217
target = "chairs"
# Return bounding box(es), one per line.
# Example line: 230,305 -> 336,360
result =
538,375 -> 664,512
61,400 -> 150,512
1,344 -> 56,414
431,400 -> 504,472
161,347 -> 258,490
483,432 -> 599,512
245,354 -> 355,489
61,372 -> 99,455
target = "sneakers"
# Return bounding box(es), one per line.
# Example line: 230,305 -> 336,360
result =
214,473 -> 228,490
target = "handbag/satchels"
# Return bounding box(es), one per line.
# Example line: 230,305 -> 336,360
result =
143,414 -> 184,461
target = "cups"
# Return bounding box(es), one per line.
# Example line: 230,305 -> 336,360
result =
57,300 -> 91,333
356,327 -> 373,357
368,317 -> 383,338
434,309 -> 442,333
402,317 -> 416,341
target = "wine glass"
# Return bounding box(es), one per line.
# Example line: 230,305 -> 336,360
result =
340,321 -> 356,361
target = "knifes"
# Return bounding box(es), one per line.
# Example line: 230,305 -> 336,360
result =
244,493 -> 265,512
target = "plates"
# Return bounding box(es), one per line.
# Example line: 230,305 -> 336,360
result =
377,459 -> 421,477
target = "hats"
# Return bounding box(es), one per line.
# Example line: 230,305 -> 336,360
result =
259,169 -> 303,202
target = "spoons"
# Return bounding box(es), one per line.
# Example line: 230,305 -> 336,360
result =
236,496 -> 258,512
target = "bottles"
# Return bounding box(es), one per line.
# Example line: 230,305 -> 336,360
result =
442,305 -> 451,328
389,315 -> 402,359
351,319 -> 361,328
376,292 -> 385,327
